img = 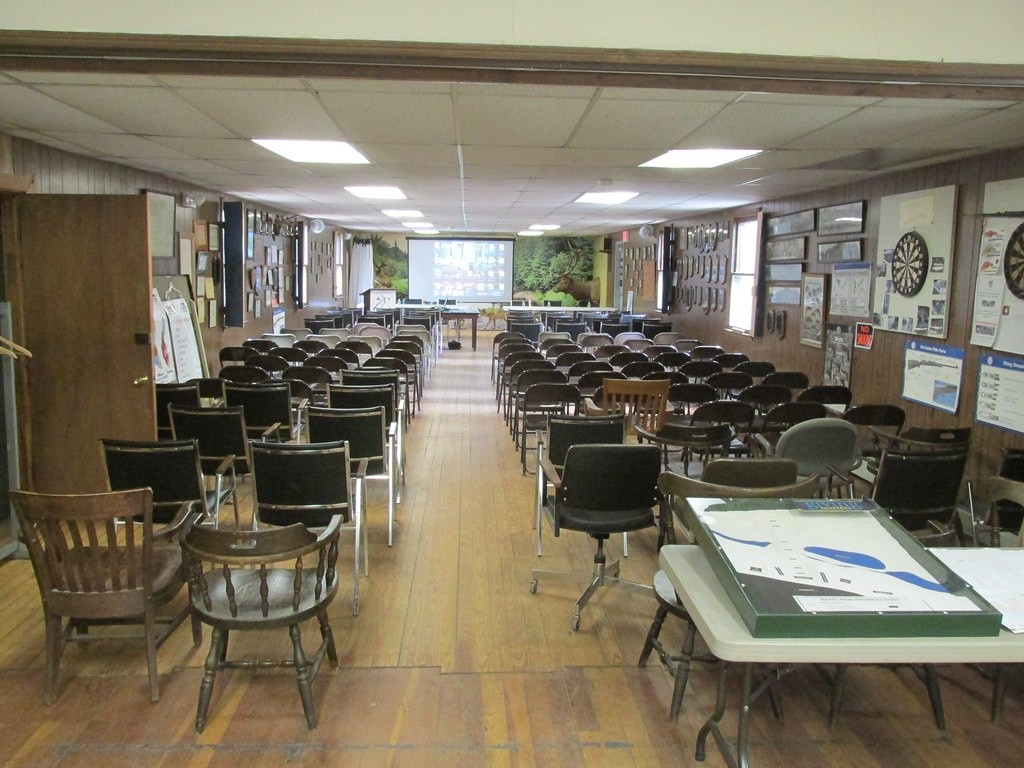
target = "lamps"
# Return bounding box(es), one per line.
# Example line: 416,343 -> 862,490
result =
308,219 -> 324,234
638,226 -> 660,240
181,191 -> 205,208
343,230 -> 352,240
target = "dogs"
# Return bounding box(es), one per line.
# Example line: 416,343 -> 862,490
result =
477,307 -> 509,331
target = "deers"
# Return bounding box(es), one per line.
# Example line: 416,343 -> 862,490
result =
373,258 -> 393,288
552,240 -> 601,303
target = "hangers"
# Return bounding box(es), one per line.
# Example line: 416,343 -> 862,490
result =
165,282 -> 181,300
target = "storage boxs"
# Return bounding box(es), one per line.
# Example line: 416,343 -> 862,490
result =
680,492 -> 1000,638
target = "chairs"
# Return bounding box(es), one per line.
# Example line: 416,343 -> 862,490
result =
9,298 -> 458,734
493,310 -> 1024,724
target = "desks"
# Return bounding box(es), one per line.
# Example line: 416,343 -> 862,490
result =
389,304 -> 476,323
502,306 -> 617,312
655,544 -> 1024,768
440,306 -> 481,351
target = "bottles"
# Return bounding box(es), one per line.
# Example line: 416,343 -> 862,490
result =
547,302 -> 551,308
522,301 -> 525,307
587,302 -> 591,308
397,299 -> 401,305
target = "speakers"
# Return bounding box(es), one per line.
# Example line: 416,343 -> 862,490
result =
604,238 -> 612,250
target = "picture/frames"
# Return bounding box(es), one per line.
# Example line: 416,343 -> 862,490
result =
817,237 -> 862,264
799,272 -> 827,349
761,264 -> 803,282
764,208 -> 816,236
762,236 -> 807,262
816,200 -> 865,236
765,284 -> 801,307
